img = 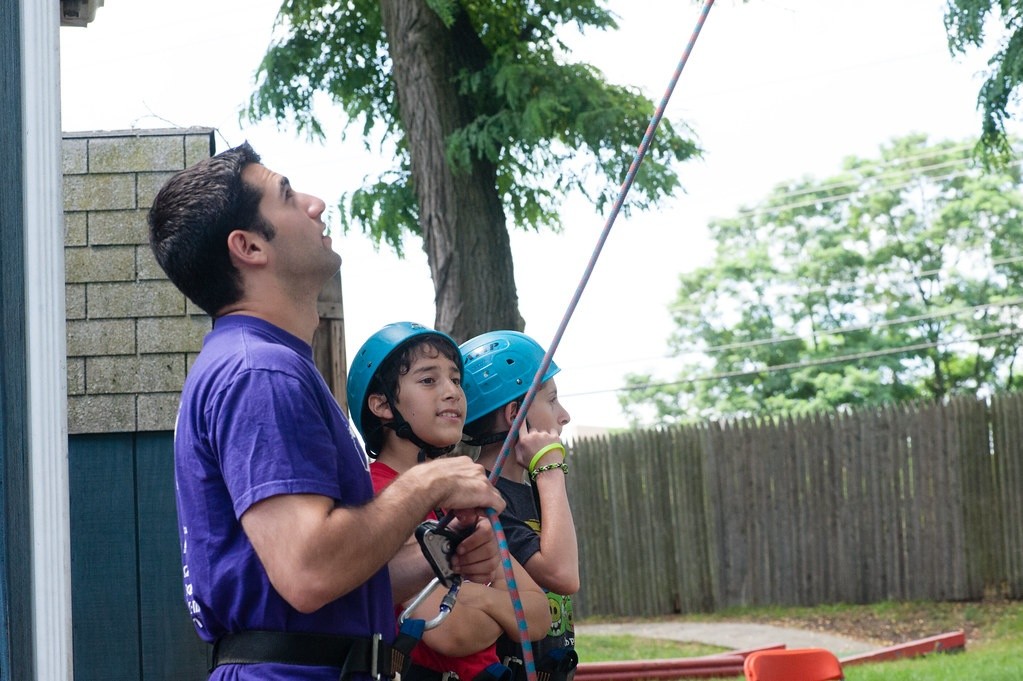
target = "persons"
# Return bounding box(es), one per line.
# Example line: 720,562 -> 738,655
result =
456,329 -> 579,681
347,323 -> 550,680
145,140 -> 506,679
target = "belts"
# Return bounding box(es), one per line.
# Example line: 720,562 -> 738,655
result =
212,629 -> 389,677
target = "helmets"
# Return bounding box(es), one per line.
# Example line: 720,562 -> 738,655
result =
459,330 -> 561,426
345,322 -> 464,444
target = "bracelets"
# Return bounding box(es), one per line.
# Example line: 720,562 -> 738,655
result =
529,442 -> 568,482
